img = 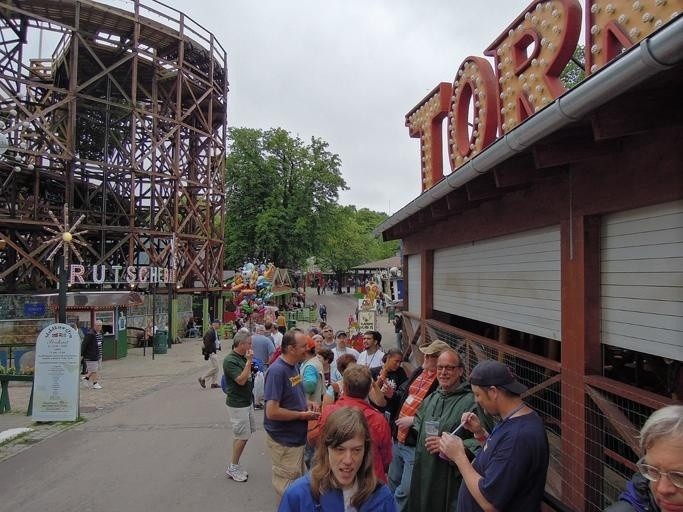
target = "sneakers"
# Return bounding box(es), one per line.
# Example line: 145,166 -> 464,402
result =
227,461 -> 248,477
255,403 -> 264,410
225,466 -> 248,482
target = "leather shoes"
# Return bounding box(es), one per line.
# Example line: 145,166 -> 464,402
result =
211,383 -> 221,388
198,376 -> 206,388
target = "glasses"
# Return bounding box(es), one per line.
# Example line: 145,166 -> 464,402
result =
439,364 -> 459,374
323,329 -> 332,333
635,454 -> 683,489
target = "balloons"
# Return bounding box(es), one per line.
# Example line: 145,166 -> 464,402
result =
228,261 -> 279,336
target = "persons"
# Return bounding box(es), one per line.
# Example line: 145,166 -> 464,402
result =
379,292 -> 387,307
66,322 -> 89,375
223,321 -> 285,482
286,289 -> 306,310
380,340 -> 550,511
264,322 -> 408,512
80,319 -> 104,390
495,332 -> 635,430
599,403 -> 682,511
319,304 -> 327,321
197,319 -> 221,390
317,278 -> 338,296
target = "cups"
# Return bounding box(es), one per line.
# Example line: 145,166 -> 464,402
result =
438,431 -> 457,462
424,419 -> 438,440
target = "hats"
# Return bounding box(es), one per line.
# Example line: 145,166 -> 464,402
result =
419,339 -> 452,355
334,330 -> 347,338
468,358 -> 528,395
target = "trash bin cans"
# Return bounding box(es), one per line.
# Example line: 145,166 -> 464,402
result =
153,330 -> 168,354
287,320 -> 296,330
387,307 -> 395,323
